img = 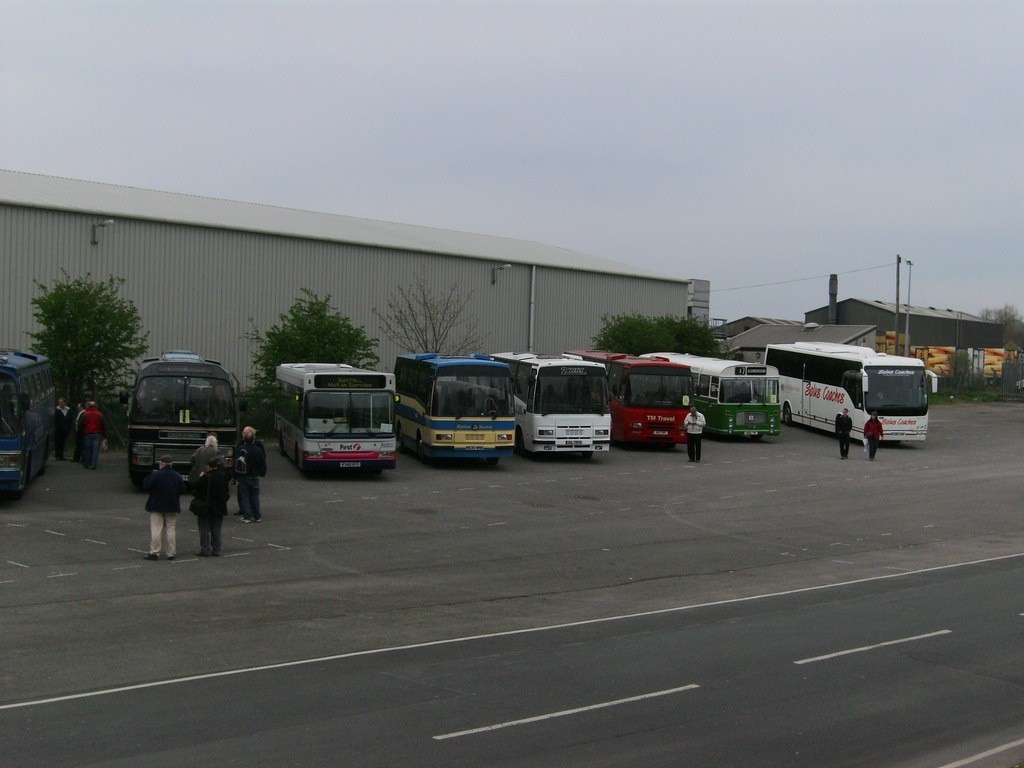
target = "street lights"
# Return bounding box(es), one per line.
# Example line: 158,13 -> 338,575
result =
904,257 -> 914,359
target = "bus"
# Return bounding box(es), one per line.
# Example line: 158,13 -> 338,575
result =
393,352 -> 522,467
638,352 -> 784,442
762,340 -> 938,447
487,350 -> 612,460
272,361 -> 402,480
562,348 -> 701,448
117,350 -> 249,491
0,347 -> 56,502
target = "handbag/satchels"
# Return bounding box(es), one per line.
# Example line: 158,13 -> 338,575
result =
863,438 -> 868,453
101,438 -> 108,451
234,453 -> 250,474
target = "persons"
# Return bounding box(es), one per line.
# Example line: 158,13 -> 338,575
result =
189,460 -> 231,557
75,401 -> 106,470
190,435 -> 224,493
864,412 -> 884,461
55,397 -> 73,460
837,408 -> 852,459
143,455 -> 184,561
232,426 -> 267,524
684,407 -> 706,463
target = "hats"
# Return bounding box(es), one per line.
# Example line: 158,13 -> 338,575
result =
157,455 -> 173,463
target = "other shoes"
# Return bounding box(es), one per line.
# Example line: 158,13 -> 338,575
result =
144,554 -> 158,560
212,550 -> 220,556
688,459 -> 694,462
237,517 -> 251,523
696,460 -> 700,463
256,516 -> 261,522
196,551 -> 209,556
169,555 -> 176,560
84,464 -> 89,468
233,511 -> 243,515
